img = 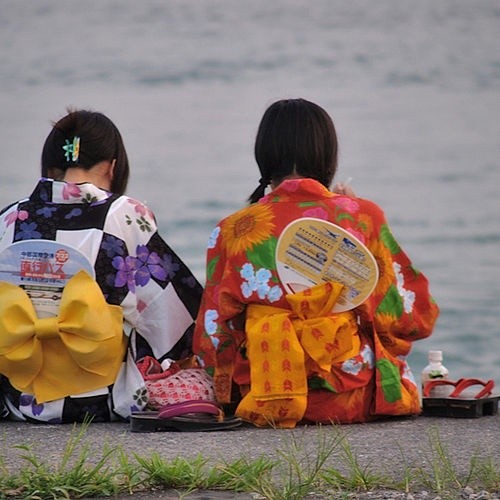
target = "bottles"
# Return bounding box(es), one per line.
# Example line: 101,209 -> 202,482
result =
421,351 -> 450,396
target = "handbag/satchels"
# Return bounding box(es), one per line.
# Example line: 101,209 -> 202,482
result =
136,356 -> 218,409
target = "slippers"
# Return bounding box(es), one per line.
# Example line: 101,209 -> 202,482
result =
422,377 -> 500,418
129,399 -> 243,433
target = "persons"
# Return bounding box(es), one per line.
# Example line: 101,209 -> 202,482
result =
0,108 -> 207,424
191,97 -> 440,430
